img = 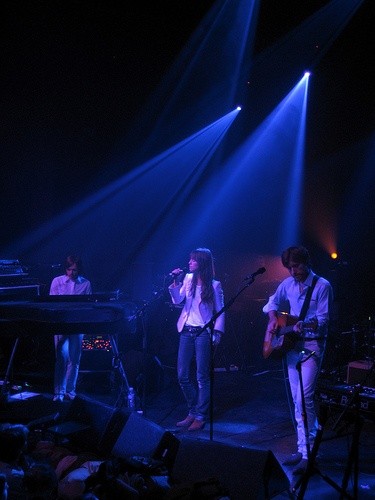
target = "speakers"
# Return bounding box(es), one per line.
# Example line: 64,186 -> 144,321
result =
167,434 -> 291,500
63,393 -> 129,449
112,411 -> 180,473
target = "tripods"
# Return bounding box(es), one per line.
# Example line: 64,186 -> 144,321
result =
292,407 -> 359,500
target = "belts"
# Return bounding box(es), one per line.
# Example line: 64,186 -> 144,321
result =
297,337 -> 324,341
183,328 -> 208,333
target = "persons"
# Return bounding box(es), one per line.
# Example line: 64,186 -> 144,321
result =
49,256 -> 92,402
0,422 -> 34,500
262,246 -> 333,476
22,464 -> 59,500
167,248 -> 225,431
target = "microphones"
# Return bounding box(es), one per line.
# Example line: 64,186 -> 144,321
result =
171,267 -> 187,279
244,266 -> 265,281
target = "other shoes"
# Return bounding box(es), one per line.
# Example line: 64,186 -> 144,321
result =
188,416 -> 208,431
52,395 -> 64,402
65,391 -> 76,400
293,455 -> 319,474
177,414 -> 195,426
288,454 -> 302,465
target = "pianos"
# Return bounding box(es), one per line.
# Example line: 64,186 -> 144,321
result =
0,288 -> 126,324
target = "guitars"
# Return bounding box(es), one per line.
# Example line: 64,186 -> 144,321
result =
263,312 -> 319,362
